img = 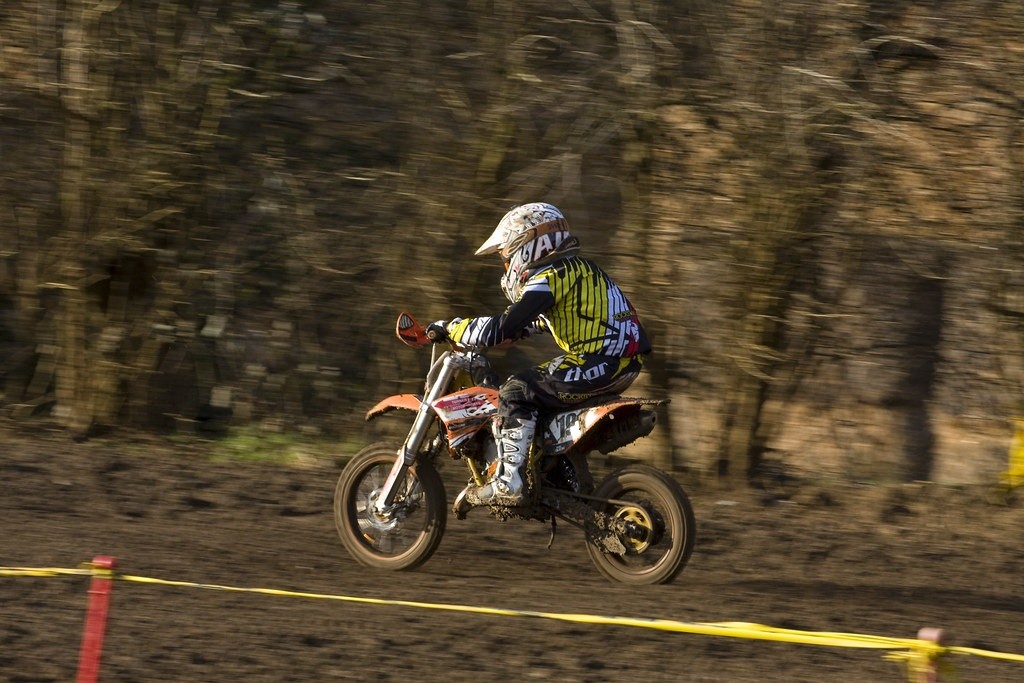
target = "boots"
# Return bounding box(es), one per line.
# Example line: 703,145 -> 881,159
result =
467,416 -> 536,506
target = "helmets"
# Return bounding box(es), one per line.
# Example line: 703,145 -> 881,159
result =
474,200 -> 579,304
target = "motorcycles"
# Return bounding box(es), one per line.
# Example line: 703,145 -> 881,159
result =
334,311 -> 695,590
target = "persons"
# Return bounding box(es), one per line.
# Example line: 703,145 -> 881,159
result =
421,200 -> 640,507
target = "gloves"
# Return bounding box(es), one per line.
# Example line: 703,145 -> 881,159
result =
427,320 -> 449,343
